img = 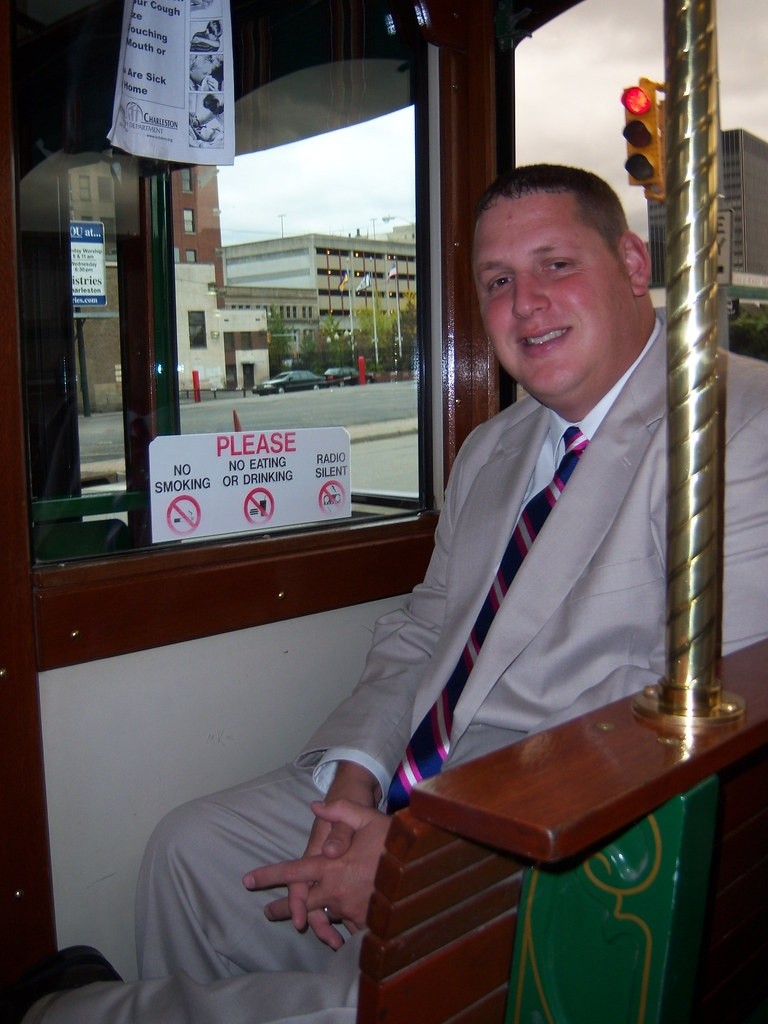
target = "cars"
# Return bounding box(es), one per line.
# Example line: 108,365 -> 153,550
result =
251,371 -> 327,397
323,367 -> 374,388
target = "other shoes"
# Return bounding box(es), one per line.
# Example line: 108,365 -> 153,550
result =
0,945 -> 125,1024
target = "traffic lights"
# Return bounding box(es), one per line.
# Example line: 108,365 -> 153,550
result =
621,78 -> 671,197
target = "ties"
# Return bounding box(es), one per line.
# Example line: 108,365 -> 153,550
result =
387,426 -> 590,814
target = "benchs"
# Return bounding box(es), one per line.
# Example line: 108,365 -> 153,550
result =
352,638 -> 768,1024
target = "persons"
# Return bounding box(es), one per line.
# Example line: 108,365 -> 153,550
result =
0,164 -> 768,1024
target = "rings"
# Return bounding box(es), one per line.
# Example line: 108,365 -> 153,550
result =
324,907 -> 331,921
